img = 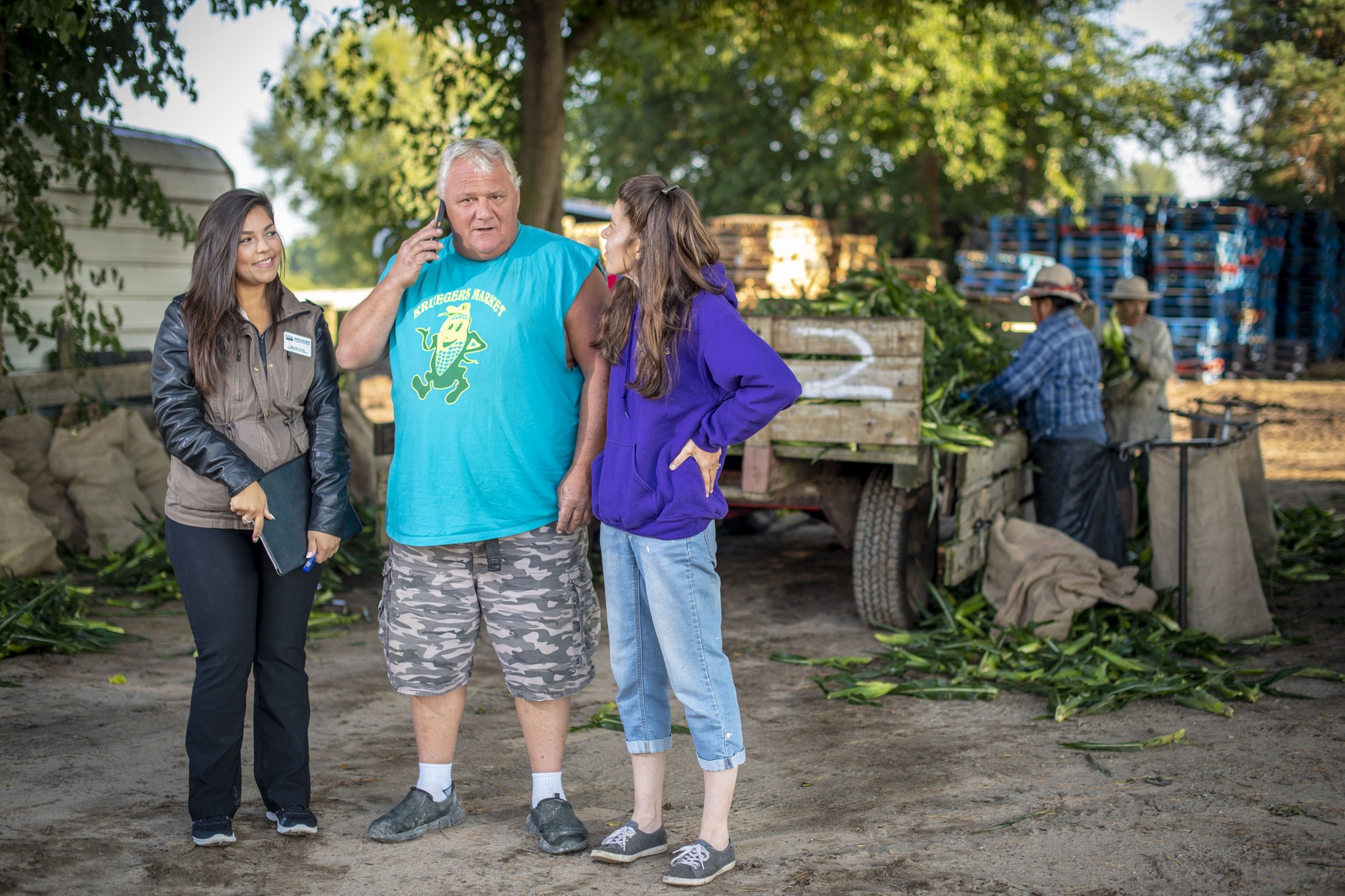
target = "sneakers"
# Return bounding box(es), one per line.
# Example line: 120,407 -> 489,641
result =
591,820 -> 668,863
267,806 -> 317,836
663,837 -> 735,885
192,815 -> 236,847
368,781 -> 466,843
526,793 -> 589,854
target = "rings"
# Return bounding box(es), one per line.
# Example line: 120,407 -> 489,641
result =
243,515 -> 252,523
715,463 -> 722,470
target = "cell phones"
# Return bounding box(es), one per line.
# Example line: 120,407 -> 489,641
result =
426,200 -> 446,264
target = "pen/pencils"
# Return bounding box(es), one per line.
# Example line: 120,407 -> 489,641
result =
302,551 -> 317,572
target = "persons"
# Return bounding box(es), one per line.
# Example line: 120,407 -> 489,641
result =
590,174 -> 802,886
150,190 -> 352,847
335,138 -> 614,854
960,265 -> 1127,632
1093,275 -> 1175,567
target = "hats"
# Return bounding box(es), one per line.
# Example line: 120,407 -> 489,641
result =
1100,276 -> 1161,299
1011,263 -> 1082,303
